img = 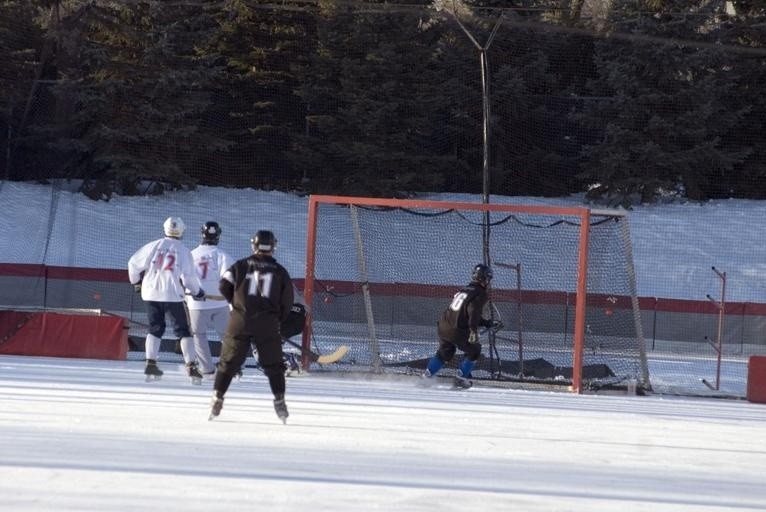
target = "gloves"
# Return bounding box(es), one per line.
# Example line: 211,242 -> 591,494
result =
192,288 -> 206,301
468,329 -> 480,343
487,319 -> 503,327
132,280 -> 142,292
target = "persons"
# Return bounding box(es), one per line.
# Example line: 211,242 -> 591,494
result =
423,264 -> 498,382
211,230 -> 294,417
180,220 -> 235,376
251,280 -> 308,371
127,216 -> 204,380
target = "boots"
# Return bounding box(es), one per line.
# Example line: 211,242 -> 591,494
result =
186,361 -> 203,378
144,359 -> 163,376
209,394 -> 224,416
453,369 -> 472,388
273,397 -> 289,418
421,370 -> 432,380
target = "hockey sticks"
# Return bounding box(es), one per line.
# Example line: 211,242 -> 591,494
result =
281,338 -> 347,364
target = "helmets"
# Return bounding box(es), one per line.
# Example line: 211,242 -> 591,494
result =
163,216 -> 185,237
251,230 -> 276,252
471,264 -> 493,281
201,221 -> 221,240
